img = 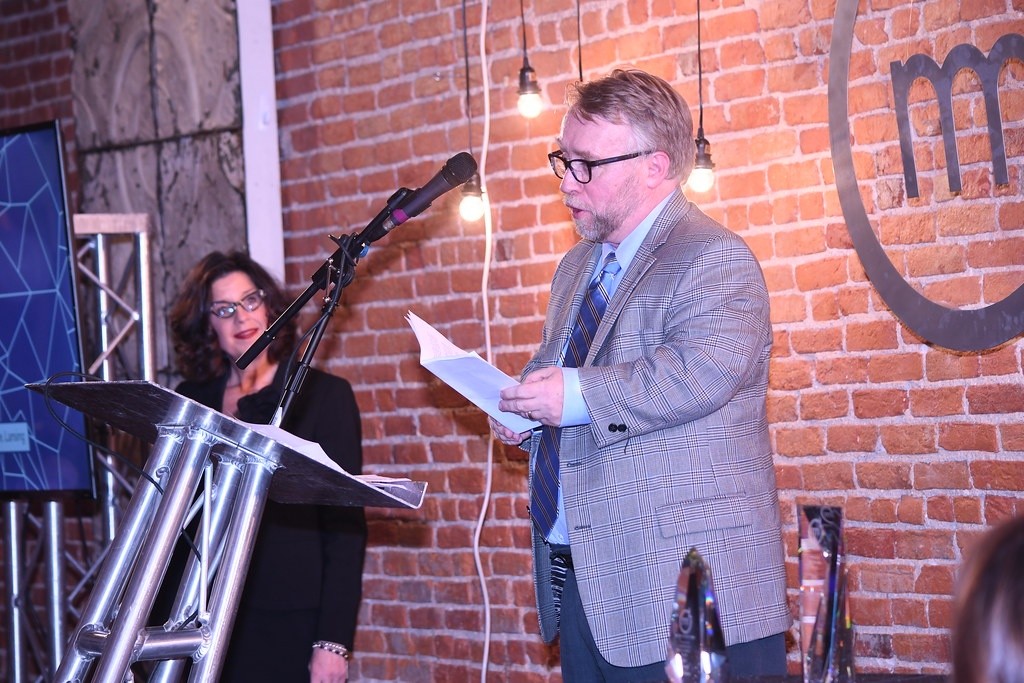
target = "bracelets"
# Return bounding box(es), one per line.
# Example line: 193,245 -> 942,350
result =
310,642 -> 349,660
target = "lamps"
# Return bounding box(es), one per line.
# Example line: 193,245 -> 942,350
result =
458,0 -> 484,223
515,0 -> 542,118
687,0 -> 715,193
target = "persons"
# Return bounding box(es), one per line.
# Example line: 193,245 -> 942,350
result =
123,250 -> 368,683
486,68 -> 794,682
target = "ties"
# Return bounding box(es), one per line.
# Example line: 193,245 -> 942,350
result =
527,246 -> 622,545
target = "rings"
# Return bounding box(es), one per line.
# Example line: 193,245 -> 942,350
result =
528,411 -> 536,421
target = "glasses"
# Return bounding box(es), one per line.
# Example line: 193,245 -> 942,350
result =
547,147 -> 652,185
207,288 -> 267,319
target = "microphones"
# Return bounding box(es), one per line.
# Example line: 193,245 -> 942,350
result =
364,151 -> 478,242
